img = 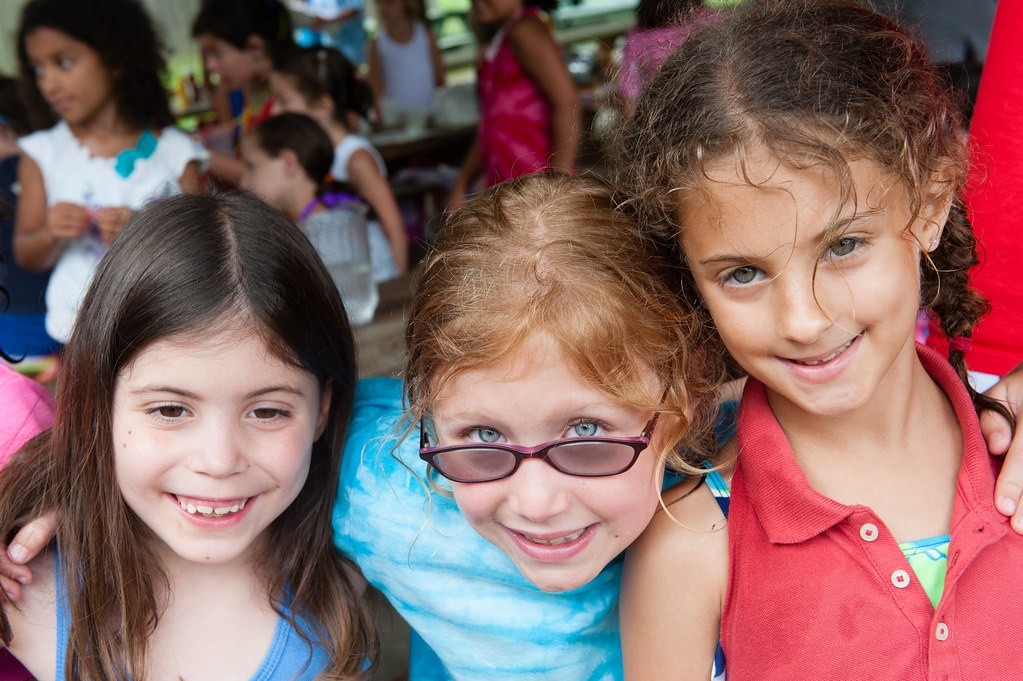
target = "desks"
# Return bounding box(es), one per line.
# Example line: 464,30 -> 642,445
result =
365,81 -> 606,200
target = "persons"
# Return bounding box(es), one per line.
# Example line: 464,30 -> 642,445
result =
0,168 -> 1022,680
607,0 -> 724,126
605,0 -> 1023,681
189,1 -> 582,325
0,0 -> 212,346
1,189 -> 379,680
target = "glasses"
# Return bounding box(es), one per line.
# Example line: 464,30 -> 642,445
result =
418,377 -> 673,484
